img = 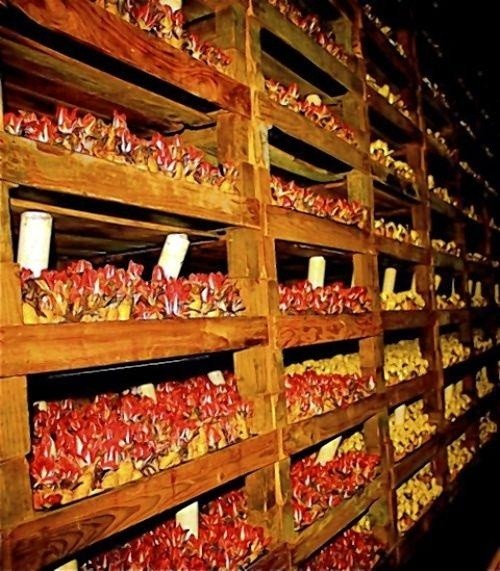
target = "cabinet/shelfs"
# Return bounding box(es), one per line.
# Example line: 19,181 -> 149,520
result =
0,0 -> 499,570
